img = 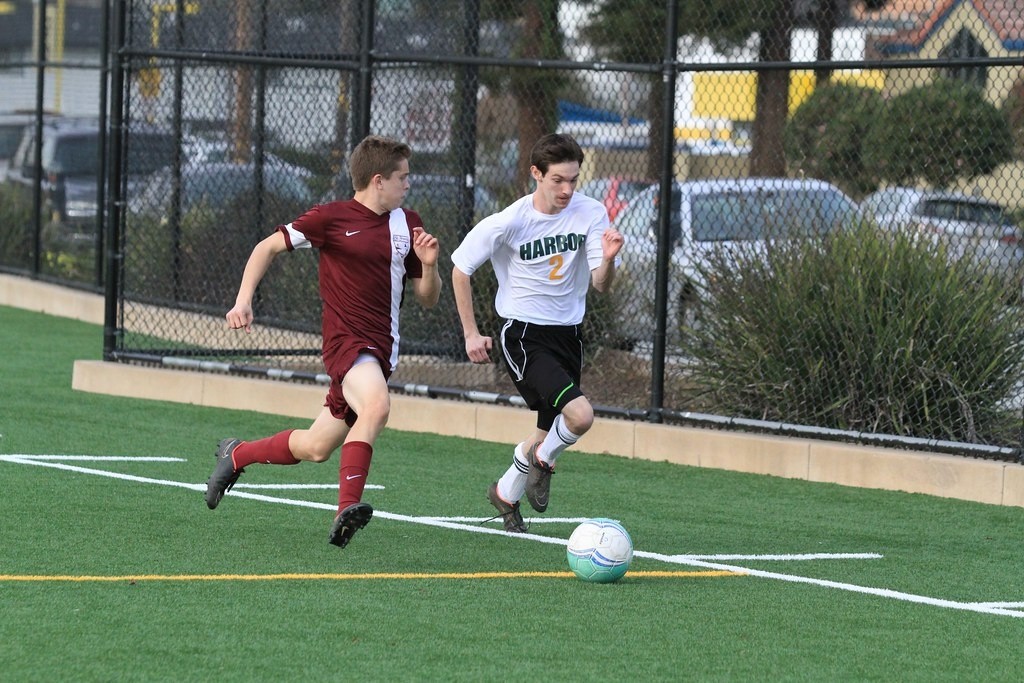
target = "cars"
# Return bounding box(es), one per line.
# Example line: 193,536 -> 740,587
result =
577,176 -> 651,223
580,175 -> 870,361
0,106 -> 328,255
858,186 -> 1024,306
321,172 -> 497,225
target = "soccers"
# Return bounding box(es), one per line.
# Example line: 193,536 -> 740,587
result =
565,517 -> 635,583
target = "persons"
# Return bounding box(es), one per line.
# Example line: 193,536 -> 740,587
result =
204,136 -> 442,547
450,133 -> 625,532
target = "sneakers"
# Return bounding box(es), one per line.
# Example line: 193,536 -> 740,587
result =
327,502 -> 373,550
526,439 -> 556,513
204,438 -> 245,510
485,480 -> 532,534
